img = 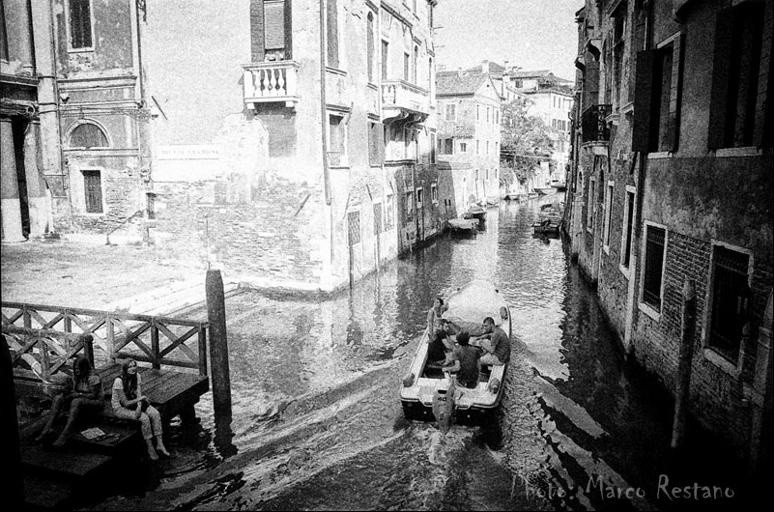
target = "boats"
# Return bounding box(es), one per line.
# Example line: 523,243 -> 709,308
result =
531,202 -> 562,234
534,188 -> 557,194
399,280 -> 512,425
446,206 -> 486,234
505,193 -> 538,200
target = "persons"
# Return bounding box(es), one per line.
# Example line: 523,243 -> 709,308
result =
36,357 -> 105,448
111,358 -> 171,461
425,298 -> 511,388
